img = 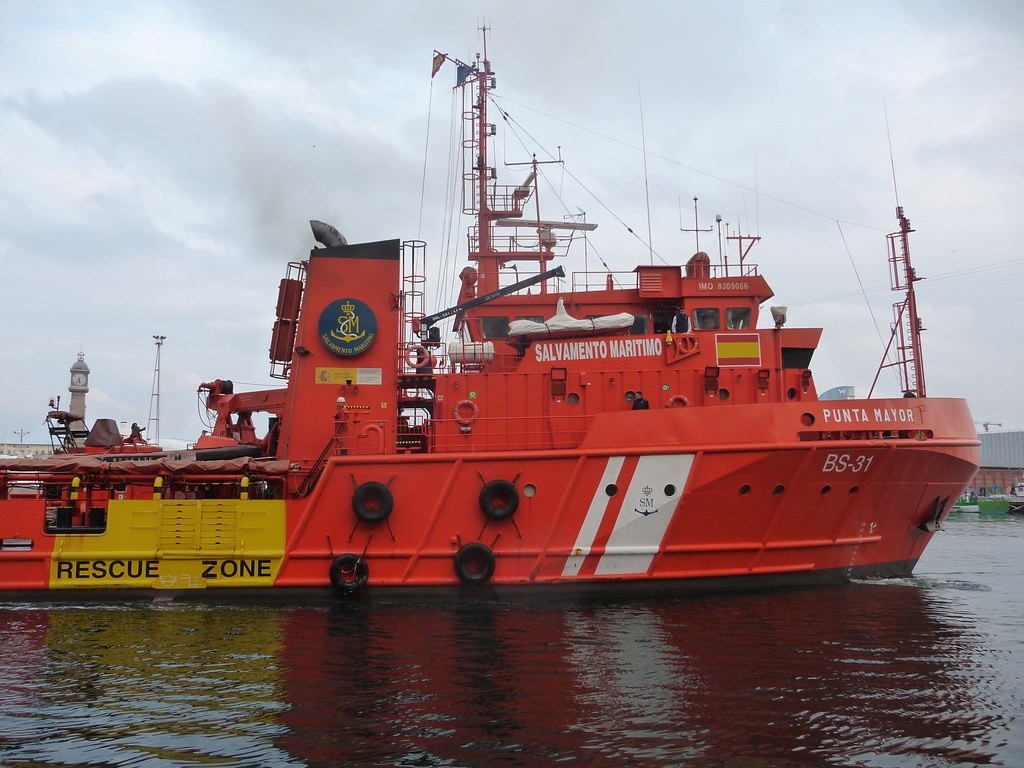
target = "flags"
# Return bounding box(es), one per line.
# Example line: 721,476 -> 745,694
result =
432,53 -> 446,77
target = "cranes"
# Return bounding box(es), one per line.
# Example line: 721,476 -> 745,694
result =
972,420 -> 1002,432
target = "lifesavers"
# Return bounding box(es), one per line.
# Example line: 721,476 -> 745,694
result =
454,400 -> 480,425
351,480 -> 394,525
665,394 -> 690,409
677,332 -> 699,354
478,479 -> 519,519
405,345 -> 430,368
329,552 -> 369,592
453,542 -> 496,583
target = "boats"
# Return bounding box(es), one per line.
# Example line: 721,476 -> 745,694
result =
0,30 -> 982,605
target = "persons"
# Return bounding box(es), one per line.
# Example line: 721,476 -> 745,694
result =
671,308 -> 691,333
333,397 -> 349,448
632,391 -> 649,410
